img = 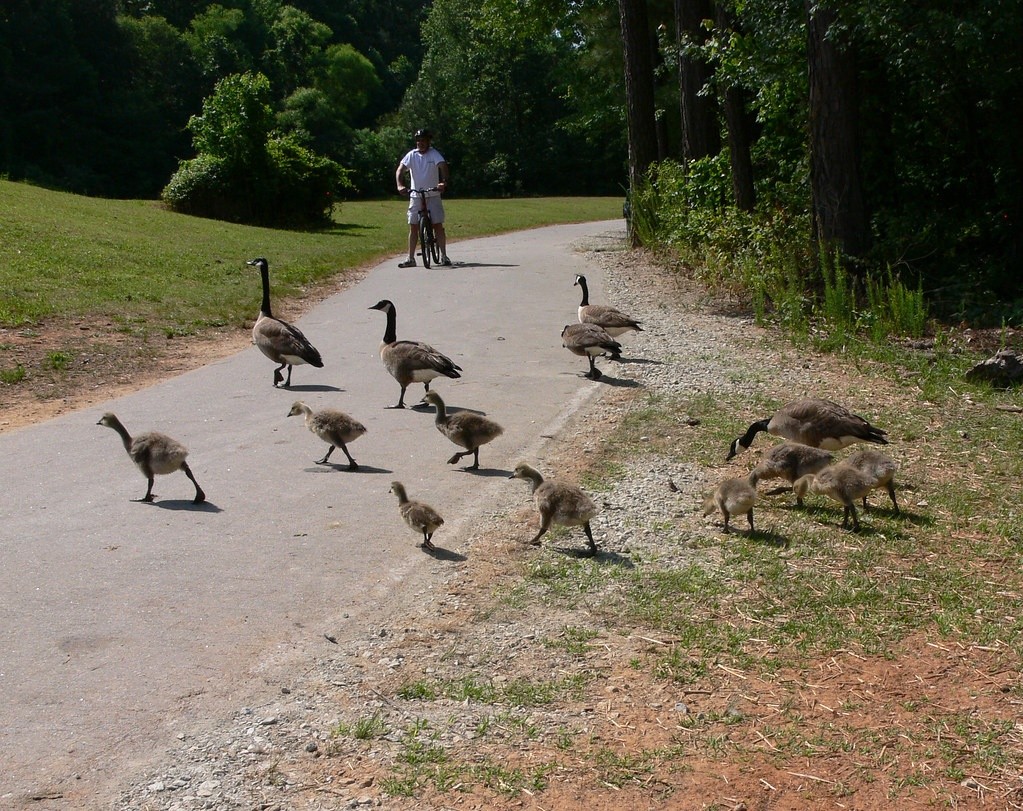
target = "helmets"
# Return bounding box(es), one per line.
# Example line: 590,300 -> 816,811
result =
414,129 -> 432,140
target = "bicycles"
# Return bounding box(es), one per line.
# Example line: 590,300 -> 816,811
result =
407,187 -> 440,268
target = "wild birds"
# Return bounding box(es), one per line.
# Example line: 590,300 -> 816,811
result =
418,390 -> 504,472
509,460 -> 598,557
246,257 -> 324,388
95,410 -> 207,507
286,400 -> 367,471
388,481 -> 444,552
560,274 -> 643,380
703,398 -> 901,534
367,299 -> 463,410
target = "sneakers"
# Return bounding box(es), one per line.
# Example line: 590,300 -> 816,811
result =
398,258 -> 416,268
440,257 -> 452,266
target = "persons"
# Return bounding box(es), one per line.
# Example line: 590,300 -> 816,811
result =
396,129 -> 451,268
